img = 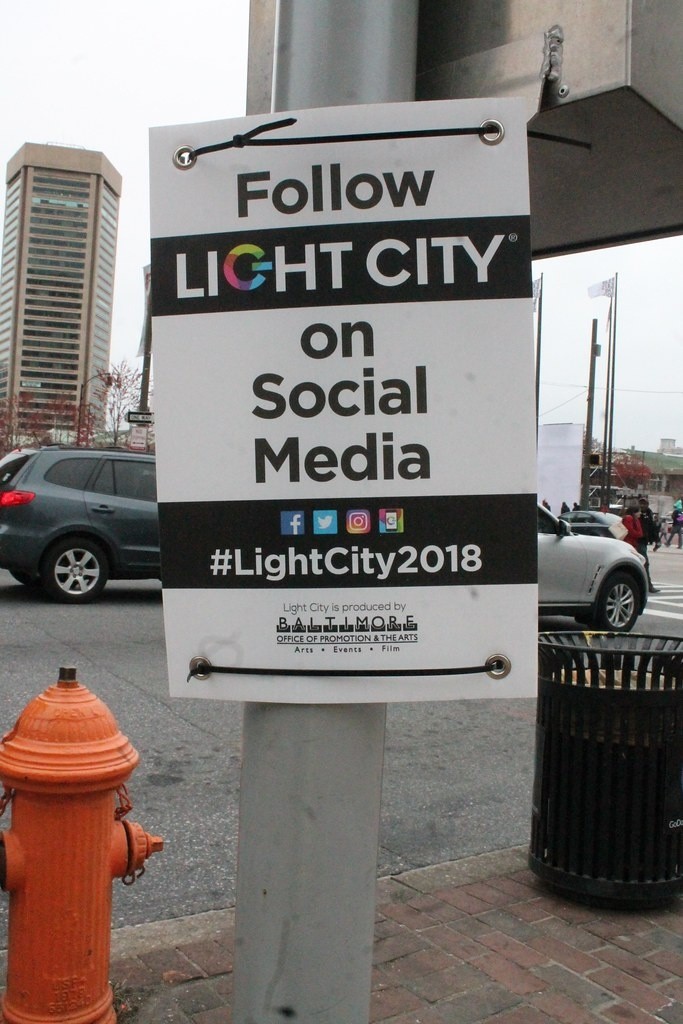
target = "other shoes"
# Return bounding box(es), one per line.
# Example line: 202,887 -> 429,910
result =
652,544 -> 661,551
665,542 -> 671,547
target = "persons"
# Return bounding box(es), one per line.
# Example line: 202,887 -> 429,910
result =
543,501 -> 551,511
561,502 -> 570,514
623,507 -> 660,593
601,503 -> 611,513
639,497 -> 661,550
665,505 -> 683,548
618,506 -> 626,516
573,503 -> 582,511
654,516 -> 668,541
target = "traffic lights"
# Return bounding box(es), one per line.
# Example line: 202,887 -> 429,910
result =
591,454 -> 603,467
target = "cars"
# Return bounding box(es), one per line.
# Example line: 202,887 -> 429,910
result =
537,503 -> 648,633
557,510 -> 624,539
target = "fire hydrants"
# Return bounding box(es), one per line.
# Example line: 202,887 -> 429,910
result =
0,665 -> 162,1024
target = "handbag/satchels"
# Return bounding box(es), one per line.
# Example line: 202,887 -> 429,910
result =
676,509 -> 683,521
608,514 -> 637,540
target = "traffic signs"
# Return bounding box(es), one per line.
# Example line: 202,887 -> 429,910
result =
127,411 -> 155,425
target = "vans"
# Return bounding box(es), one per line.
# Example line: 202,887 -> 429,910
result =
1,445 -> 162,602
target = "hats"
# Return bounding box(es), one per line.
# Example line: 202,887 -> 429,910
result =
638,497 -> 650,504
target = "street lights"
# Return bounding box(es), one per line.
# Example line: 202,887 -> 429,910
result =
76,372 -> 113,445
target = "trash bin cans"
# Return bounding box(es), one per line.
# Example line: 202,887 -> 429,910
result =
528,630 -> 683,911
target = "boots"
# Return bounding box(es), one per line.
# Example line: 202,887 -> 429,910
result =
648,576 -> 660,593
